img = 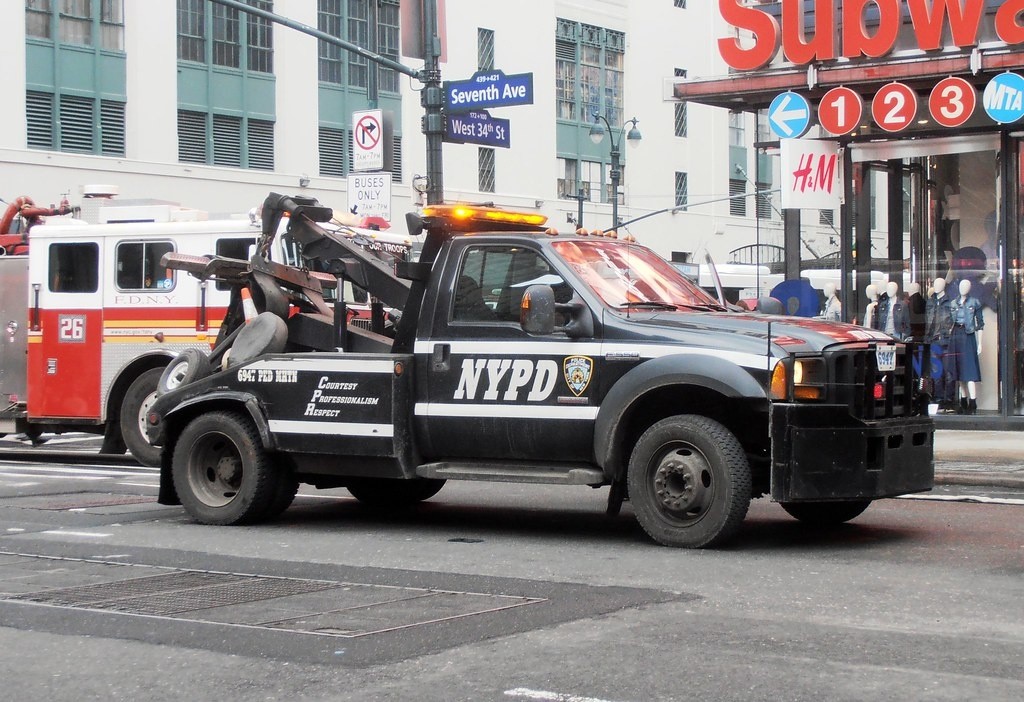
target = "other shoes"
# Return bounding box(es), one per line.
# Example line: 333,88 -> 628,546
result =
935,400 -> 945,414
946,400 -> 955,413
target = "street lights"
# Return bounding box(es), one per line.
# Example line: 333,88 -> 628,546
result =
588,111 -> 643,231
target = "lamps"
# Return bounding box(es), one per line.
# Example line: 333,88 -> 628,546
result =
535,200 -> 544,207
299,178 -> 311,187
673,209 -> 679,214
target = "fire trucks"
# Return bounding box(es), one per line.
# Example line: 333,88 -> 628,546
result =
0,185 -> 414,467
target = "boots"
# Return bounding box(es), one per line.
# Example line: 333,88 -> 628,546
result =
957,397 -> 977,415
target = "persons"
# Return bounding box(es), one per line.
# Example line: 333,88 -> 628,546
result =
863,278 -> 985,415
823,282 -> 841,321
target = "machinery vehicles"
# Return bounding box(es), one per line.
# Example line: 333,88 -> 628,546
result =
144,191 -> 937,550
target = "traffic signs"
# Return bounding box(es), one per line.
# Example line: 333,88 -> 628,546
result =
424,67 -> 533,149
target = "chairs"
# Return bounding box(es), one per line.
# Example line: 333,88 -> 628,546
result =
452,275 -> 500,322
